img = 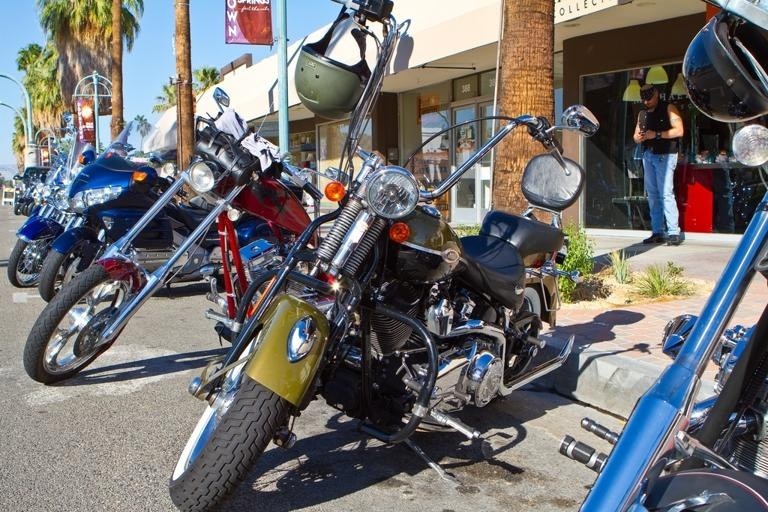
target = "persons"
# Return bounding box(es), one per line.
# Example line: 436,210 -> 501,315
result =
633,83 -> 684,246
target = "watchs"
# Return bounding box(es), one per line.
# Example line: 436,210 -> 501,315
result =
656,130 -> 661,140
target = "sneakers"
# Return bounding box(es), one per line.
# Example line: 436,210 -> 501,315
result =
667,235 -> 682,245
643,235 -> 663,243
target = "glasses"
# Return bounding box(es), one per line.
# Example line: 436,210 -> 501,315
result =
642,94 -> 652,102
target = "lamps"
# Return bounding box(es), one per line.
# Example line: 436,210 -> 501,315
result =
621,66 -> 689,102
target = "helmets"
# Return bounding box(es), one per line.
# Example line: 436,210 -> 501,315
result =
683,9 -> 768,123
294,43 -> 372,122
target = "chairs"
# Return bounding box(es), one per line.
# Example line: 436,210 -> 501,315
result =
610,158 -> 650,230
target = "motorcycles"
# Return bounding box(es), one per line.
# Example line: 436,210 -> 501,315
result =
576,1 -> 766,512
167,1 -> 602,511
